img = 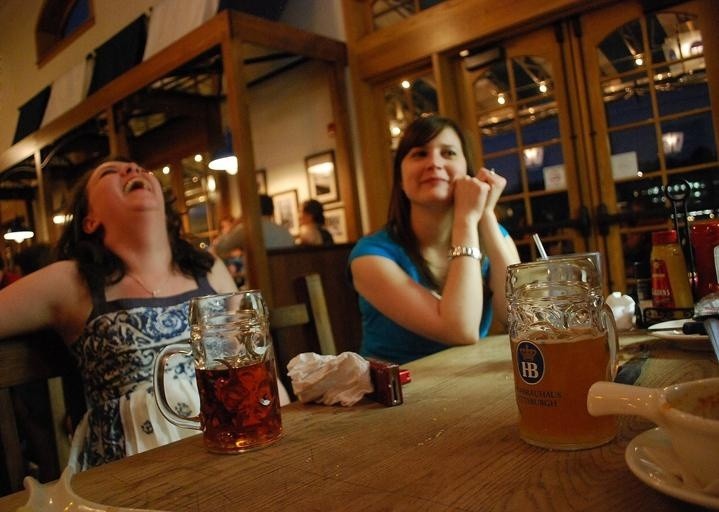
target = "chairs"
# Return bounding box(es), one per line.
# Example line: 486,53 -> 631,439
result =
270,275 -> 337,405
0,334 -> 82,490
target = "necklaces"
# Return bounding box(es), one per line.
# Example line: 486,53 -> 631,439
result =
127,268 -> 183,296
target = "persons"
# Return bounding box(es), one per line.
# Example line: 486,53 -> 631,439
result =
293,199 -> 334,248
346,116 -> 521,367
210,194 -> 293,255
2,159 -> 291,473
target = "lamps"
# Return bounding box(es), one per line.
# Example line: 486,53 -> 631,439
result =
0,216 -> 34,243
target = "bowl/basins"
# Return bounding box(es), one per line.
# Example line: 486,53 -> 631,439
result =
586,370 -> 718,497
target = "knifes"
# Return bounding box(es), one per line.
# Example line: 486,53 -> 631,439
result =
646,322 -> 708,335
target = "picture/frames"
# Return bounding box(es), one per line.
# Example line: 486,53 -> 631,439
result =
256,170 -> 267,195
324,206 -> 349,244
271,189 -> 301,238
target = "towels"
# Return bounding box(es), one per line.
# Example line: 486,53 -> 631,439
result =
603,290 -> 635,333
285,353 -> 372,410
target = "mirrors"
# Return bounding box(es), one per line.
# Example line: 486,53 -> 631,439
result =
304,150 -> 338,202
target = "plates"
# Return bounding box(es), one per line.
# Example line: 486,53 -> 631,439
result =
648,317 -> 713,353
624,426 -> 719,508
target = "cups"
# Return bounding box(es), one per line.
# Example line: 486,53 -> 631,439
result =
503,252 -> 622,452
153,290 -> 286,456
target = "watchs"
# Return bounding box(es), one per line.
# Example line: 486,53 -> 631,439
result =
447,243 -> 484,262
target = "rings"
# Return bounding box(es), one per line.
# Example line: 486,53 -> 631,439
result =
490,167 -> 496,175
